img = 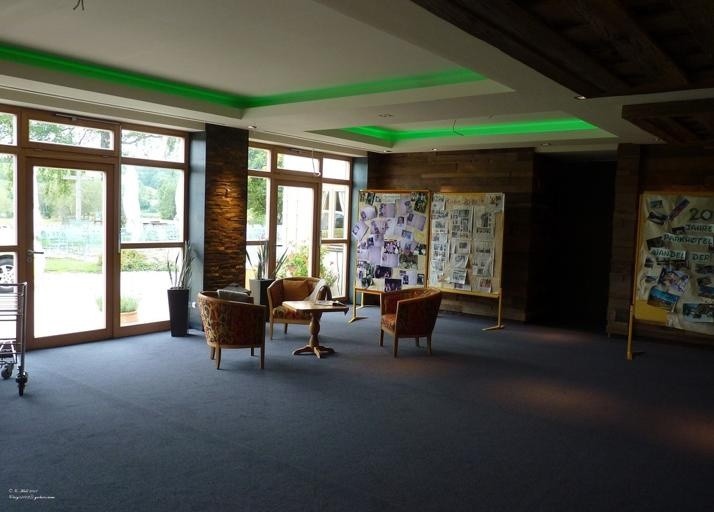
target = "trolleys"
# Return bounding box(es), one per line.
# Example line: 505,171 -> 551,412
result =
0,282 -> 28,396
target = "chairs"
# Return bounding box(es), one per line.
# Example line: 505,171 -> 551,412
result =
196,277 -> 327,370
380,287 -> 443,359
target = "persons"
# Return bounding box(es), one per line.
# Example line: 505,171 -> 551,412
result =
384,240 -> 399,255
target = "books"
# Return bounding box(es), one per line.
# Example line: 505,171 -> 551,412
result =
314,299 -> 347,306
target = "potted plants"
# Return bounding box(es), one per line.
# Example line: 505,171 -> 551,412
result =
244,241 -> 289,322
96,295 -> 139,327
166,240 -> 196,337
